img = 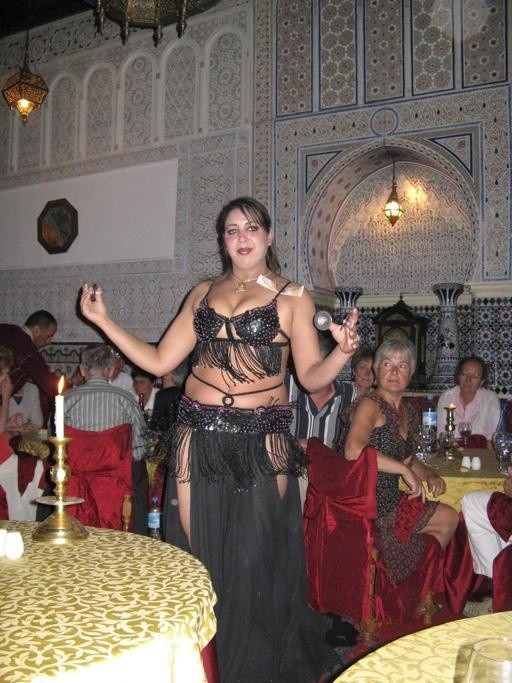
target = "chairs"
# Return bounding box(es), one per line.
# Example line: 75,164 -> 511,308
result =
58,422 -> 141,540
482,488 -> 512,616
299,437 -> 447,647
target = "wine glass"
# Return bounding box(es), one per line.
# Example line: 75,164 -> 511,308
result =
418,428 -> 435,464
495,435 -> 506,471
458,421 -> 473,455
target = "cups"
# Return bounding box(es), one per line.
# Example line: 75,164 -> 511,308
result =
438,431 -> 454,449
504,432 -> 512,453
466,636 -> 511,682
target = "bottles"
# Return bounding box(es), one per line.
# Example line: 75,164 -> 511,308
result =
1,527 -> 25,561
461,456 -> 480,470
149,496 -> 162,537
492,399 -> 511,459
420,395 -> 436,449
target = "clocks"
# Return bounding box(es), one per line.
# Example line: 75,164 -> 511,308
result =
372,289 -> 429,388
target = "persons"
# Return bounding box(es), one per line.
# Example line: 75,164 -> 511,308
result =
339,346 -> 380,418
459,460 -> 512,601
129,366 -> 167,422
77,195 -> 362,683
108,347 -> 134,390
343,324 -> 459,620
434,351 -> 502,448
1,346 -> 26,433
287,326 -> 355,464
55,343 -> 154,536
1,308 -> 70,425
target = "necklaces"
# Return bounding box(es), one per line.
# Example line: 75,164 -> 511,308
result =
222,270 -> 273,292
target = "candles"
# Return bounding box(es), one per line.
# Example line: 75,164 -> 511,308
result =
55,373 -> 68,440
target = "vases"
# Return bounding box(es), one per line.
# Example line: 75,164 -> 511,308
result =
428,282 -> 464,390
331,282 -> 365,380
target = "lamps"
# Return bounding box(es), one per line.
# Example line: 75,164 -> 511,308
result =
94,0 -> 217,44
382,155 -> 407,225
442,402 -> 459,461
1,0 -> 52,124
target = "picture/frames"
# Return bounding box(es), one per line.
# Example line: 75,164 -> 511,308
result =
36,196 -> 77,254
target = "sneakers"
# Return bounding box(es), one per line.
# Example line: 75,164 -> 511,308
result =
463,597 -> 494,618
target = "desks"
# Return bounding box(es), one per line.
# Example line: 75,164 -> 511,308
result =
0,510 -> 221,680
6,428 -> 165,501
380,444 -> 507,617
332,606 -> 512,683
